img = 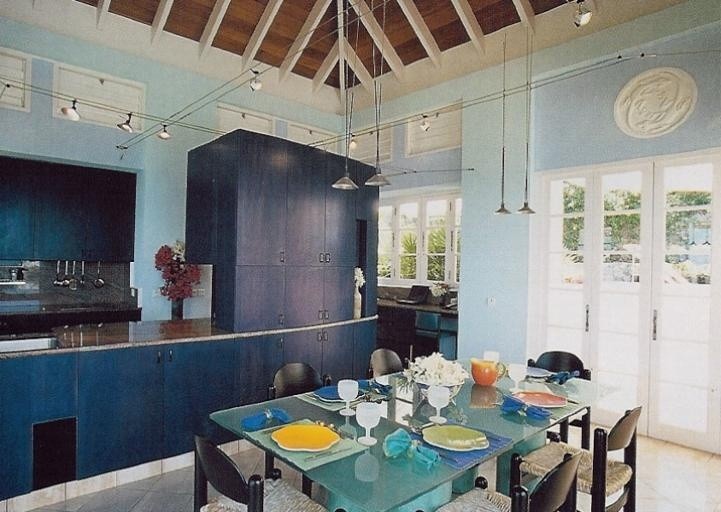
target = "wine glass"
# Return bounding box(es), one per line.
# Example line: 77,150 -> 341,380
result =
354,443 -> 379,483
426,386 -> 449,423
337,380 -> 358,416
507,364 -> 526,393
337,417 -> 356,441
355,403 -> 380,446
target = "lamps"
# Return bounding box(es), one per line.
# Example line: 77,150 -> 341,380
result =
566,0 -> 594,29
493,32 -> 513,216
330,0 -> 359,192
245,65 -> 267,95
365,1 -> 393,187
59,96 -> 171,142
515,22 -> 537,216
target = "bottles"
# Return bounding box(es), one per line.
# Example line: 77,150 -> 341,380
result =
10,269 -> 18,281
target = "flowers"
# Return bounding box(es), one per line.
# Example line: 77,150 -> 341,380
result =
431,280 -> 451,296
352,265 -> 366,289
392,352 -> 472,386
154,236 -> 204,299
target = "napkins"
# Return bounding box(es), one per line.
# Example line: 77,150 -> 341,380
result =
499,395 -> 551,421
240,409 -> 292,432
358,379 -> 392,396
405,421 -> 514,471
546,366 -> 584,386
383,428 -> 442,471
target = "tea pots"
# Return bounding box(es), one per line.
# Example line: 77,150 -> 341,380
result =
470,358 -> 506,387
467,382 -> 505,408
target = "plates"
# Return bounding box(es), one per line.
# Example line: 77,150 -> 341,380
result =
313,386 -> 364,402
421,425 -> 489,452
512,391 -> 567,408
270,424 -> 340,452
526,366 -> 550,378
413,379 -> 464,387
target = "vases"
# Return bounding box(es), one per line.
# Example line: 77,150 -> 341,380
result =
170,296 -> 186,319
352,288 -> 362,320
416,381 -> 466,406
440,294 -> 449,305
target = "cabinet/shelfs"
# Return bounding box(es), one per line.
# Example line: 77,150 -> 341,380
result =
285,324 -> 353,386
184,127 -> 381,333
235,334 -> 283,406
79,332 -> 235,496
354,321 -> 379,379
1,348 -> 80,512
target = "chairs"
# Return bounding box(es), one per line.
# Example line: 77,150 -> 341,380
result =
528,351 -> 591,451
194,436 -> 344,511
417,451 -> 582,512
264,364 -> 332,497
368,349 -> 409,380
510,406 -> 643,512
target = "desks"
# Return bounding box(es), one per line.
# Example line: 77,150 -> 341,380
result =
378,298 -> 456,361
208,358 -> 590,511
